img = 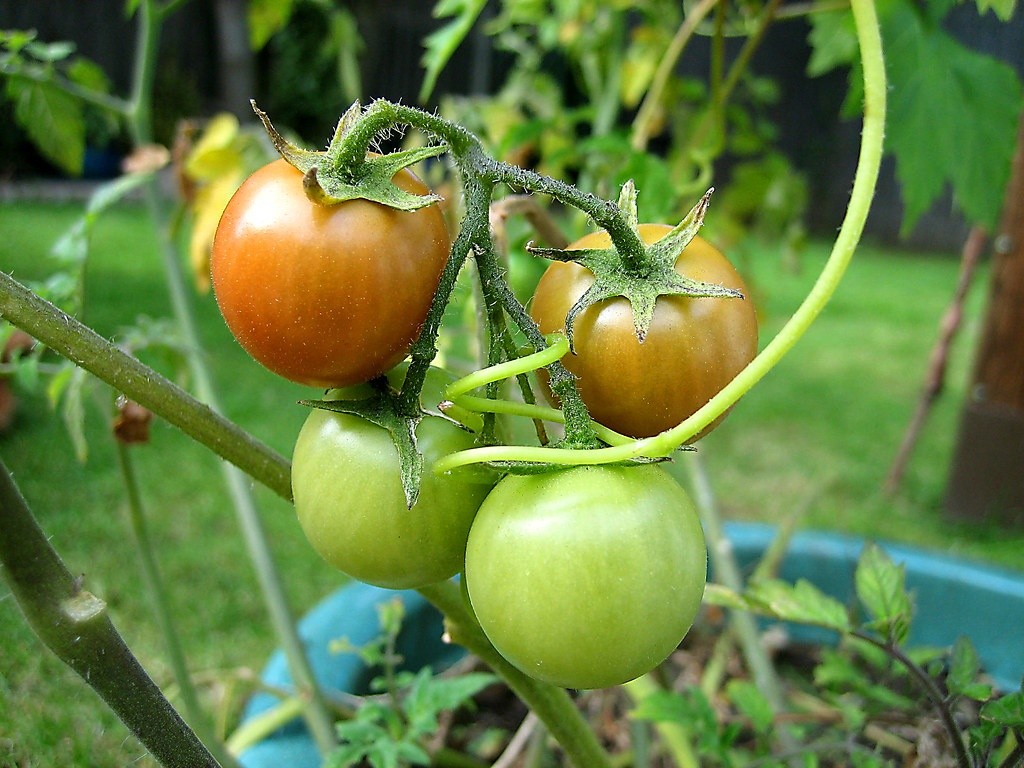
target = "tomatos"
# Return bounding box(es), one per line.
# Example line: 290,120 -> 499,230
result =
209,150 -> 759,690
